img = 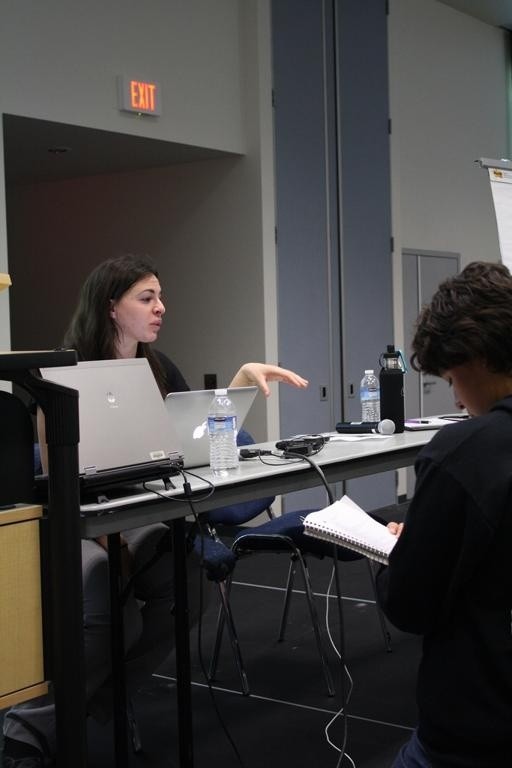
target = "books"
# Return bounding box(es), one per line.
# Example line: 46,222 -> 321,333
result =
404,418 -> 468,431
302,494 -> 399,566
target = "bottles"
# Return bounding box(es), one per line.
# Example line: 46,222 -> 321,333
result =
360,370 -> 381,422
379,345 -> 407,434
207,389 -> 240,470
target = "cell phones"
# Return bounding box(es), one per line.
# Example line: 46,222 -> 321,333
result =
276,440 -> 323,450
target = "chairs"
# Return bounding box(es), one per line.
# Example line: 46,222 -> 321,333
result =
190,427 -> 391,700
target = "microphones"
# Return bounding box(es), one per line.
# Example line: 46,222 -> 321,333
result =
238,448 -> 271,458
336,418 -> 396,435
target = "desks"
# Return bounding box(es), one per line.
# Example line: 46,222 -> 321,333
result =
80,410 -> 472,767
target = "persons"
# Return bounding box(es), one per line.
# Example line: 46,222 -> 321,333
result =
375,260 -> 512,768
1,256 -> 310,768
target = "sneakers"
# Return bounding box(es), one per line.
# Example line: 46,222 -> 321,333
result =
89,684 -> 114,727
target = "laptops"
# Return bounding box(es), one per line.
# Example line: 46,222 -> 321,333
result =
32,357 -> 186,496
164,385 -> 259,470
